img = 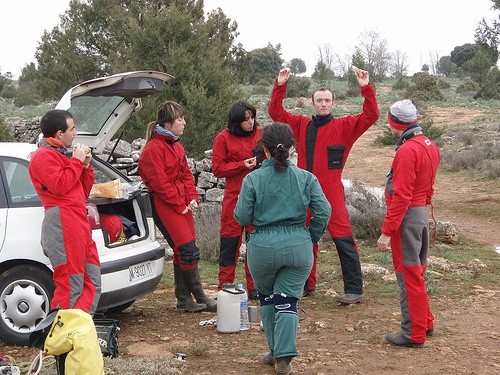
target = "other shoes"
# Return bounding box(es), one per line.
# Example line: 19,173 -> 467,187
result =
249,290 -> 258,299
302,291 -> 316,299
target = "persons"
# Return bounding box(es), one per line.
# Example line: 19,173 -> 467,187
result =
377,98 -> 442,348
211,101 -> 267,301
137,101 -> 217,312
232,122 -> 332,375
268,68 -> 380,304
28,109 -> 103,318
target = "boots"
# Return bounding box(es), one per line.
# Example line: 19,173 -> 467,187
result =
174,264 -> 207,312
182,266 -> 217,311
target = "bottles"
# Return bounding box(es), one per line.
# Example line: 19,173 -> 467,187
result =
217,282 -> 245,333
238,284 -> 251,330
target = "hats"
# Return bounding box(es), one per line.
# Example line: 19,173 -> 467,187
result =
387,99 -> 418,131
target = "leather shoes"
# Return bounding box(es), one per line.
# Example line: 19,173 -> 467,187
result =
275,360 -> 292,375
385,332 -> 424,348
426,329 -> 435,336
335,293 -> 363,304
263,350 -> 274,364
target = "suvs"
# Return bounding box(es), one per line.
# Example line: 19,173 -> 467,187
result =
0,70 -> 176,346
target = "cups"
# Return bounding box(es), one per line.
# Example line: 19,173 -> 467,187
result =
248,306 -> 258,322
